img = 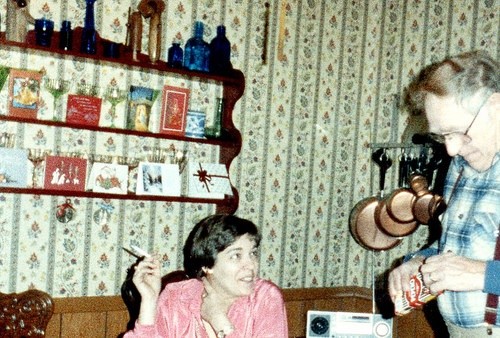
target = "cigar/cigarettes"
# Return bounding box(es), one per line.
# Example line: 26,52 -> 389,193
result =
129,245 -> 152,258
123,215 -> 289,338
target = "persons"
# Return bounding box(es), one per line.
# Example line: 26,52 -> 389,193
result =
390,51 -> 500,338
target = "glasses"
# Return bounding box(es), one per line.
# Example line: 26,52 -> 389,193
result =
430,98 -> 490,144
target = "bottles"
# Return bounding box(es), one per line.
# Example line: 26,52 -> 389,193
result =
81,0 -> 98,54
167,38 -> 184,68
184,20 -> 211,72
210,25 -> 230,75
59,20 -> 74,51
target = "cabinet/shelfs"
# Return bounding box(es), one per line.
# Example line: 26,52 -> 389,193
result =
0,26 -> 246,215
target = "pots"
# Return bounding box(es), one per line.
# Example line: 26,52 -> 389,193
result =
406,148 -> 442,226
348,148 -> 403,256
387,148 -> 419,224
374,147 -> 420,237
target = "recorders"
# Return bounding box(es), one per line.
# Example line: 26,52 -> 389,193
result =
306,310 -> 393,338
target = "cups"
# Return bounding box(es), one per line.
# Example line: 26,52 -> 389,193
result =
186,110 -> 206,138
34,18 -> 54,49
101,41 -> 123,58
